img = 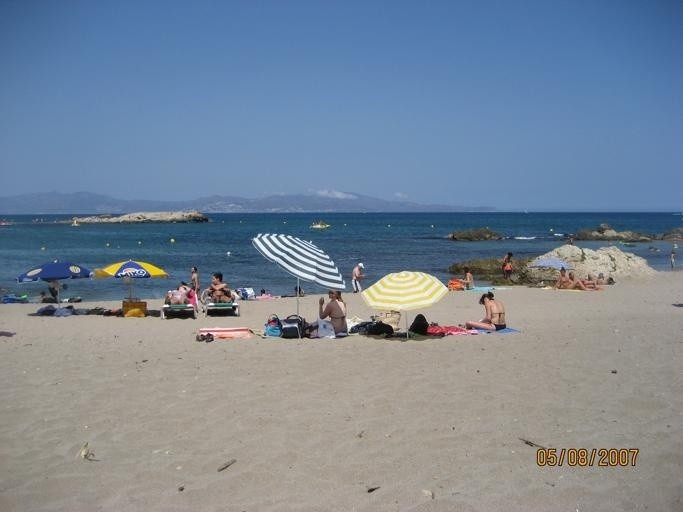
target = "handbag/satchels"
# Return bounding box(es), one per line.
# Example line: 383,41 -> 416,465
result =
264,313 -> 283,337
282,314 -> 306,338
367,321 -> 393,338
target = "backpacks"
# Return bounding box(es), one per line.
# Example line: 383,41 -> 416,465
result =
409,313 -> 429,336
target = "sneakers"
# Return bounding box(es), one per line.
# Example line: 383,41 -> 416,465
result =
195,333 -> 214,342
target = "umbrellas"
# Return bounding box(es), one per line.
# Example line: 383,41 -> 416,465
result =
94,259 -> 168,301
14,258 -> 92,311
250,234 -> 348,317
360,270 -> 449,340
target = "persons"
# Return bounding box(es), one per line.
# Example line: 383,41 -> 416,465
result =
39,292 -> 46,302
502,261 -> 512,278
46,279 -> 57,299
669,251 -> 678,269
585,273 -> 616,285
317,289 -> 347,334
457,291 -> 506,332
189,266 -> 203,300
501,251 -> 512,279
556,267 -> 593,291
260,287 -> 265,295
567,271 -> 596,289
164,281 -> 192,305
207,271 -> 235,304
454,266 -> 473,290
673,240 -> 678,249
350,262 -> 365,292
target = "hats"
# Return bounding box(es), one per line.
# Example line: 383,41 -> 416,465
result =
358,262 -> 364,269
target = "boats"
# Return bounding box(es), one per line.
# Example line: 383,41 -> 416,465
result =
311,224 -> 331,229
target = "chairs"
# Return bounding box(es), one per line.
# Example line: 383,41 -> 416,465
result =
161,302 -> 240,319
